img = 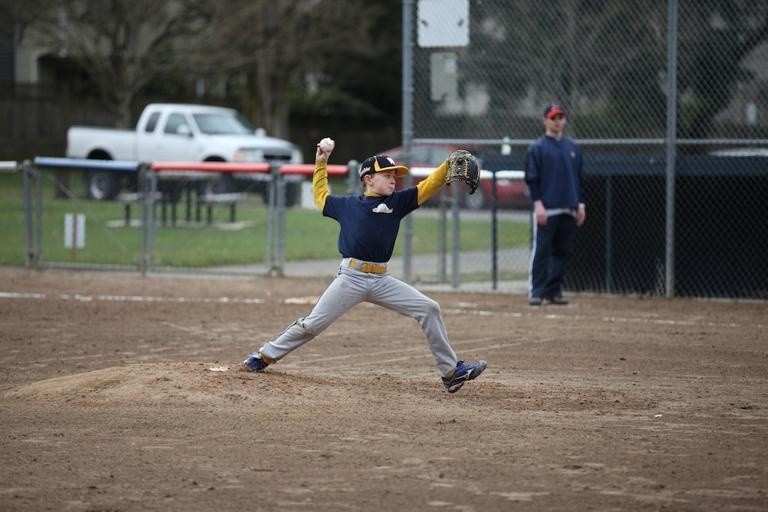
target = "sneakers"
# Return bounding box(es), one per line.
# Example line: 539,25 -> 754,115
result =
440,359 -> 488,394
527,295 -> 570,306
241,351 -> 273,373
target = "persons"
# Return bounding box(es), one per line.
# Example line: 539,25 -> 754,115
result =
525,104 -> 586,306
242,139 -> 487,393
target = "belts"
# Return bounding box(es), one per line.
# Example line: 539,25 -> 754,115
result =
347,257 -> 387,275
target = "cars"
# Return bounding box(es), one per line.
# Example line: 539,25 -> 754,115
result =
366,140 -> 538,210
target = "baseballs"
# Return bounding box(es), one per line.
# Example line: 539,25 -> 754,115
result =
320,138 -> 335,151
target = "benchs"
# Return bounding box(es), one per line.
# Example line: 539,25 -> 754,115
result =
107,166 -> 254,231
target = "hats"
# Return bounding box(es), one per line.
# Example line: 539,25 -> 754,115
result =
542,103 -> 565,119
355,154 -> 411,183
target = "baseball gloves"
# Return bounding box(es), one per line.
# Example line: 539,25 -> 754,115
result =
445,150 -> 480,194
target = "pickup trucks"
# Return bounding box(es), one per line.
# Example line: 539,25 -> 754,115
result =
62,101 -> 308,212
